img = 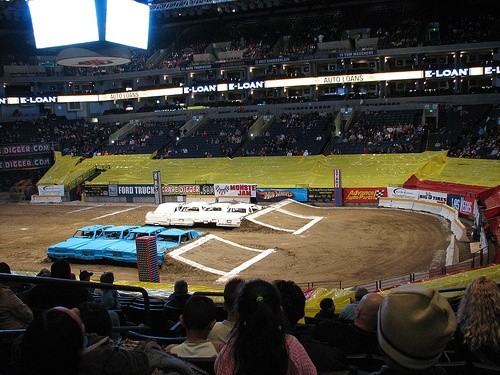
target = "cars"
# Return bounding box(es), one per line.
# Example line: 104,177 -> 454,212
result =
47,224 -> 203,269
143,201 -> 260,229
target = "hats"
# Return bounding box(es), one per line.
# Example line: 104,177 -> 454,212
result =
174,280 -> 188,293
100,271 -> 114,283
36,267 -> 50,276
320,298 -> 332,309
79,270 -> 94,281
50,259 -> 71,279
377,283 -> 459,369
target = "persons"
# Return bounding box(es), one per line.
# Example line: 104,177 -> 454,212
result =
0,0 -> 500,162
369,283 -> 458,375
0,260 -> 500,375
214,279 -> 317,375
